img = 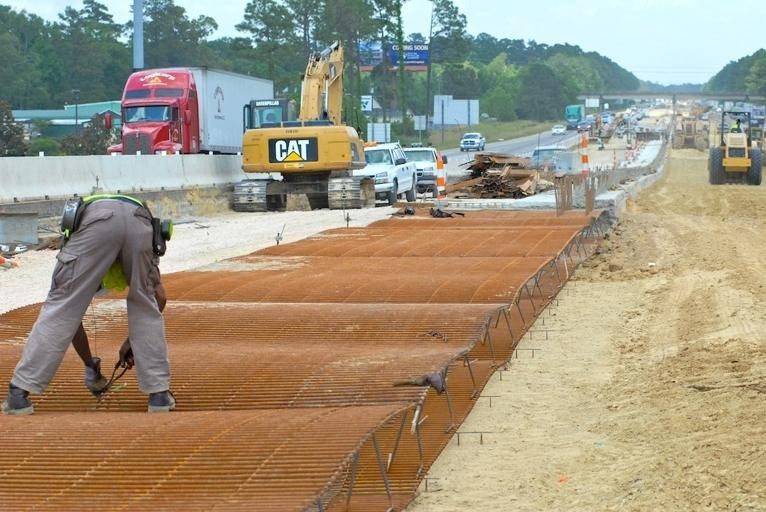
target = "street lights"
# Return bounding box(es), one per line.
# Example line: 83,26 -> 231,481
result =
71,89 -> 80,133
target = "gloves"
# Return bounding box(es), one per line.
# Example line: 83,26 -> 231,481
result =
84,356 -> 111,402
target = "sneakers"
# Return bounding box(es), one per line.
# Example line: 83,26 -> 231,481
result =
2,391 -> 35,414
146,390 -> 177,411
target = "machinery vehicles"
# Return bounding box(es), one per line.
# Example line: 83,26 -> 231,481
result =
709,110 -> 762,186
232,40 -> 377,211
672,105 -> 713,151
745,126 -> 766,165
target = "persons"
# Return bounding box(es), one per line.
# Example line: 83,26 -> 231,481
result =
0,194 -> 176,413
730,118 -> 741,133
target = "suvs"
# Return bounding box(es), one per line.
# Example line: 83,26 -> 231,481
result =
352,139 -> 418,206
400,143 -> 448,194
460,132 -> 486,151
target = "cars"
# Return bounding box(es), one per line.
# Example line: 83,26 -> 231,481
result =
717,124 -> 729,133
751,110 -> 765,123
551,125 -> 568,135
585,101 -> 669,140
577,120 -> 593,133
531,146 -> 573,172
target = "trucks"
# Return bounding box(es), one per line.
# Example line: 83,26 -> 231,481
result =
106,67 -> 275,156
564,104 -> 586,129
